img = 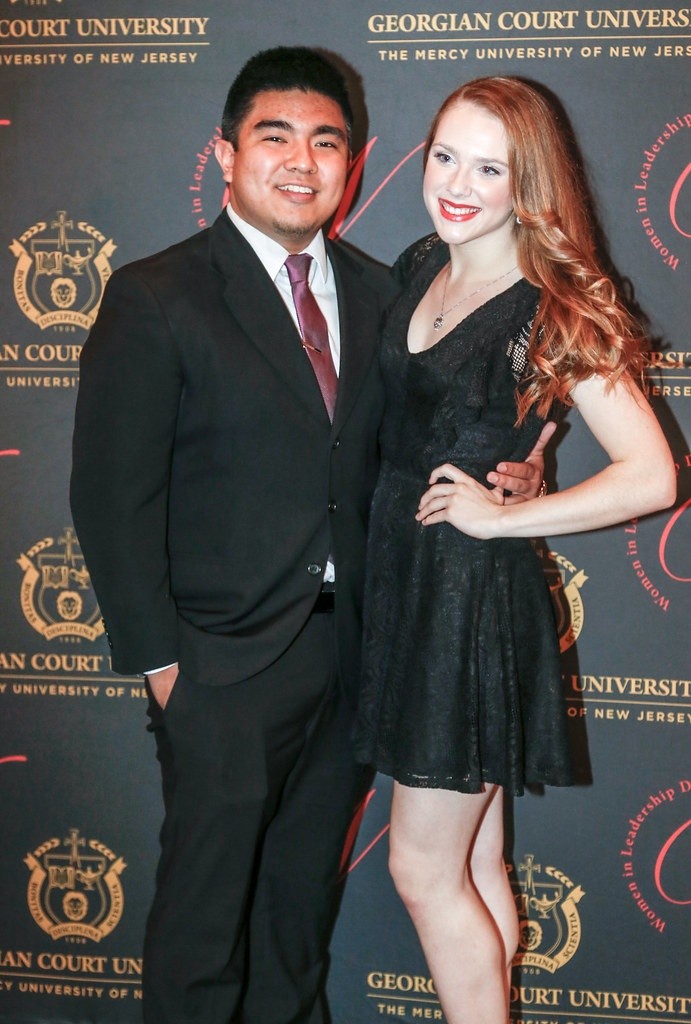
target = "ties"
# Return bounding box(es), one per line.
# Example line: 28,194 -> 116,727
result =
285,253 -> 340,427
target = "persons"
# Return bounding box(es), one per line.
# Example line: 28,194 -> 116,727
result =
346,74 -> 676,1024
69,45 -> 557,1024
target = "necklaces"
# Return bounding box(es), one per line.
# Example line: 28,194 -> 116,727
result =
434,262 -> 519,330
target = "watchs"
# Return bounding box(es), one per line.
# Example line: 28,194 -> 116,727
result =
538,479 -> 547,497
